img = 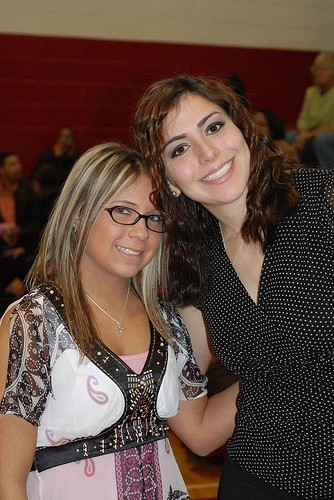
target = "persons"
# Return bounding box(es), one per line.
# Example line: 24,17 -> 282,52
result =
223,49 -> 334,169
0,127 -> 79,319
136,75 -> 333,499
1,141 -> 242,500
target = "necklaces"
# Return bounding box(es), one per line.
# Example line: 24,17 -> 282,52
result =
218,221 -> 244,267
84,277 -> 131,336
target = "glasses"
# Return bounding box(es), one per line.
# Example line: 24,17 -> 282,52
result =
103,205 -> 167,233
